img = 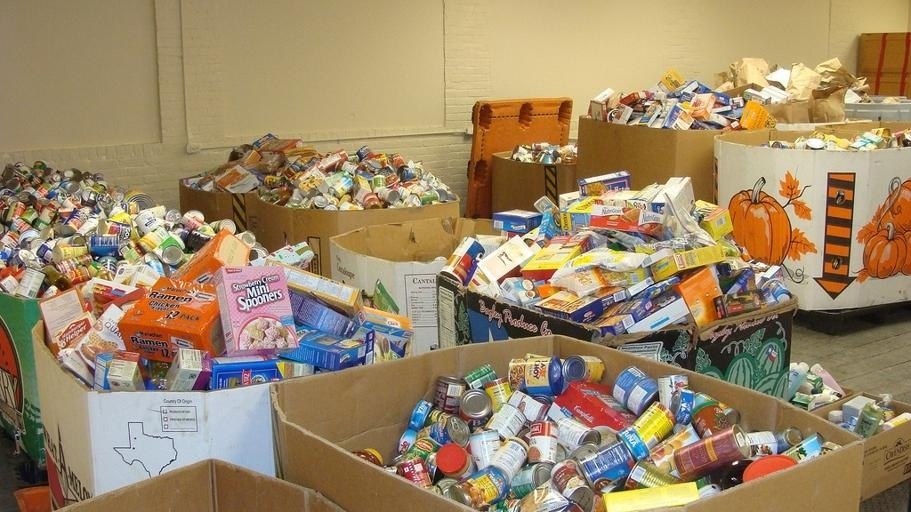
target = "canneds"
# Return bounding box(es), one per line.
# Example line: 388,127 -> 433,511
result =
669,390 -> 695,425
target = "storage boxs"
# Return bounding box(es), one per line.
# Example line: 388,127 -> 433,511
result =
326,216 -> 499,356
435,271 -> 797,402
51,459 -> 347,512
271,331 -> 866,512
809,392 -> 911,502
491,149 -> 576,217
31,304 -> 414,504
179,176 -> 260,236
712,119 -> 910,313
577,111 -> 716,205
2,291 -> 43,474
804,387 -> 859,413
257,196 -> 461,279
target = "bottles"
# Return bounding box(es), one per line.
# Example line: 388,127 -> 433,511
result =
397,429 -> 419,456
162,246 -> 190,270
562,357 -> 606,383
122,247 -> 142,268
418,416 -> 471,450
413,191 -> 440,205
36,244 -> 54,263
0,161 -> 123,208
135,212 -> 160,235
349,447 -> 383,467
16,267 -> 45,300
328,176 -> 352,198
364,195 -> 382,210
440,237 -> 485,283
746,431 -> 777,457
19,207 -> 38,226
483,377 -> 513,409
529,423 -> 558,465
463,363 -> 498,390
692,402 -> 731,439
6,202 -> 25,226
511,464 -> 552,498
88,235 -> 119,257
489,439 -> 529,480
7,250 -> 36,271
507,391 -> 550,423
508,357 -> 564,398
556,418 -> 600,448
397,461 -> 432,492
742,455 -> 795,481
409,399 -> 453,430
810,363 -> 845,396
578,442 -> 635,490
673,424 -> 751,481
437,446 -> 477,480
458,389 -> 492,431
98,257 -> 117,276
435,377 -> 466,414
235,231 -> 256,247
61,211 -> 87,237
625,460 -> 681,491
378,188 -> 399,205
178,229 -> 212,253
33,208 -> 55,232
448,469 -> 509,508
52,243 -> 88,264
779,432 -> 825,464
612,367 -> 659,414
64,267 -> 90,285
484,404 -> 527,440
633,402 -> 675,449
659,375 -> 689,409
551,462 -> 593,512
211,219 -> 236,236
10,219 -> 32,235
775,428 -> 802,454
373,176 -> 386,194
786,362 -> 810,401
469,432 -> 500,470
